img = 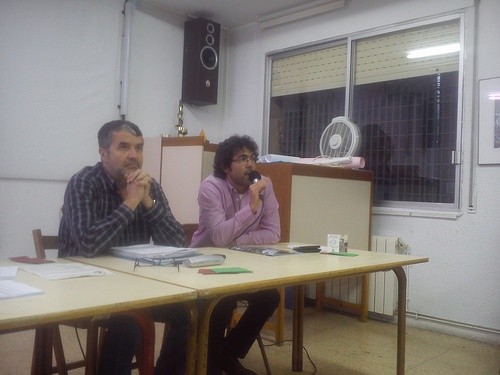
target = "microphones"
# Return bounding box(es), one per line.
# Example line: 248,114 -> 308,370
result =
248,170 -> 264,195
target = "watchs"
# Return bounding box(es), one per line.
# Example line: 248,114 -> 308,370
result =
153,200 -> 157,205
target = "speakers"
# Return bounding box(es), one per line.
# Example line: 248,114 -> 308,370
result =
183,17 -> 220,105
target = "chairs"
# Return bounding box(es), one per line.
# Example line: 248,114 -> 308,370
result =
183,223 -> 273,375
31,229 -> 128,375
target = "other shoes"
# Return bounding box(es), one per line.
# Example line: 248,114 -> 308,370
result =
221,340 -> 257,375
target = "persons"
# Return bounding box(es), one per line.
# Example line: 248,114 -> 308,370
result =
188,134 -> 281,375
56,120 -> 191,375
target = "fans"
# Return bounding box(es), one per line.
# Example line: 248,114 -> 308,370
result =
319,116 -> 361,158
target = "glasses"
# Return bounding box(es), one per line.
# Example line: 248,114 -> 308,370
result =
230,155 -> 258,163
133,256 -> 179,272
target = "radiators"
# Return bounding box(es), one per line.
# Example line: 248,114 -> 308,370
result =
324,236 -> 406,317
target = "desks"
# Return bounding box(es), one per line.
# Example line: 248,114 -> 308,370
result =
0,240 -> 428,375
159,135 -> 374,323
